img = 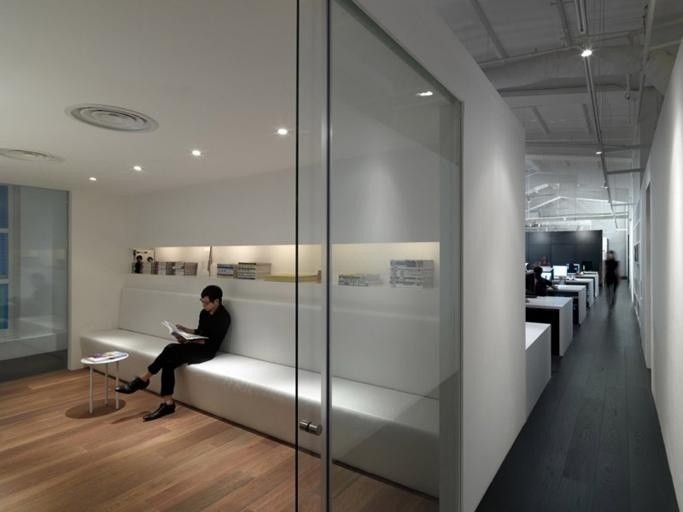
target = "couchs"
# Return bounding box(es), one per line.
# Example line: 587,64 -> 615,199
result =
81,288 -> 438,502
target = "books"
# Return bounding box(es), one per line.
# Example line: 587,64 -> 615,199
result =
151,260 -> 435,288
161,320 -> 209,343
88,350 -> 127,362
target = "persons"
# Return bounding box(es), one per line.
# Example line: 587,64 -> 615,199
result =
9,273 -> 58,318
525,254 -> 580,297
134,255 -> 144,273
116,285 -> 231,421
604,250 -> 619,305
146,257 -> 153,273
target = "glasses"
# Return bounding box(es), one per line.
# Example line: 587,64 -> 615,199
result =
200,298 -> 213,306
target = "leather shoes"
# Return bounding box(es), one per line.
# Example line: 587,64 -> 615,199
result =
143,399 -> 176,420
114,376 -> 149,393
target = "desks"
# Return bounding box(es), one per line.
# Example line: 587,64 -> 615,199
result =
81,352 -> 128,415
526,271 -> 600,418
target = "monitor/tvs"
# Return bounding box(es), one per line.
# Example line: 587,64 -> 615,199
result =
525,262 -> 585,284
